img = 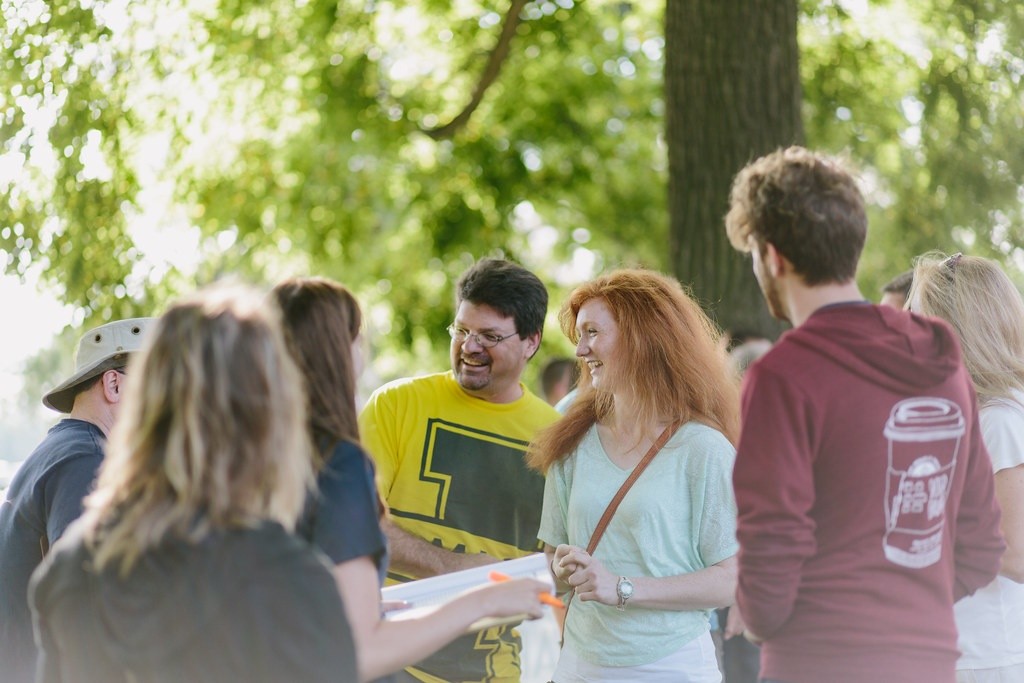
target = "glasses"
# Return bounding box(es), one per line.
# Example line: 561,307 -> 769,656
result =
447,319 -> 520,349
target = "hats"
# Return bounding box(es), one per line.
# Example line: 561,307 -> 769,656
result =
42,315 -> 162,413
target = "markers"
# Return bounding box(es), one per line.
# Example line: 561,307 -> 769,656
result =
487,569 -> 568,610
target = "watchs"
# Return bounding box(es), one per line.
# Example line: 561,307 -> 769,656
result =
616,576 -> 634,611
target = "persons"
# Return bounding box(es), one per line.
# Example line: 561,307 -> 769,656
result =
0,249 -> 1024,683
724,147 -> 1007,683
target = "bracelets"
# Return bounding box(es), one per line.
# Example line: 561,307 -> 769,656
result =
549,557 -> 556,577
